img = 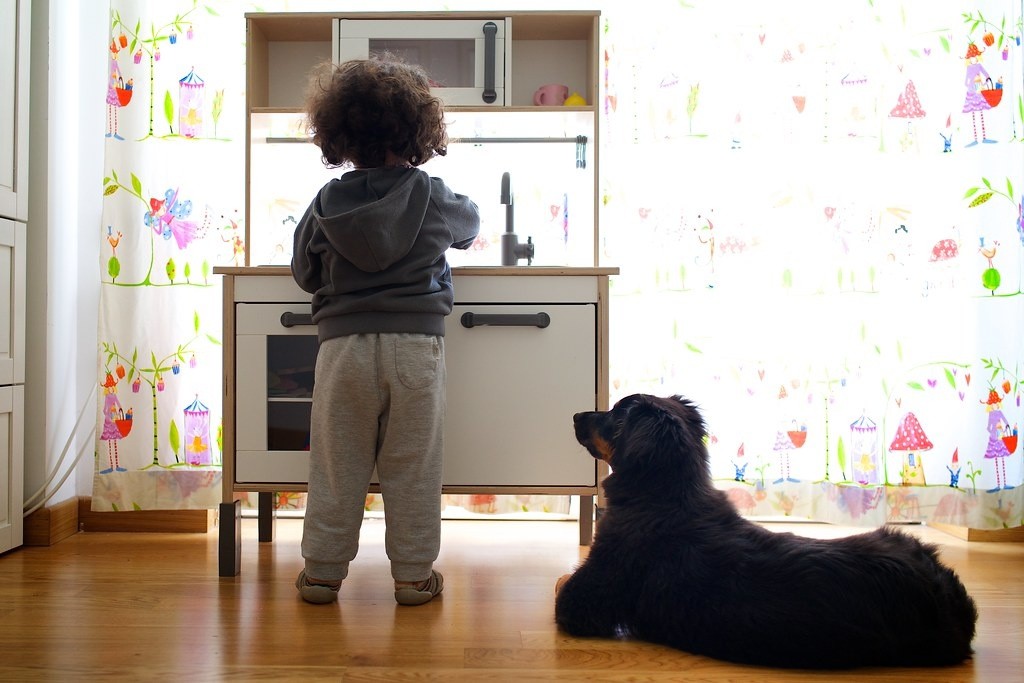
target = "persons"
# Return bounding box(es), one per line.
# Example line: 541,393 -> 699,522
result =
291,50 -> 480,605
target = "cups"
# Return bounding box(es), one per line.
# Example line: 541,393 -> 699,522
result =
534,84 -> 569,106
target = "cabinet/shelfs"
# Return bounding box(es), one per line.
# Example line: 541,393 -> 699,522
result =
212,10 -> 619,576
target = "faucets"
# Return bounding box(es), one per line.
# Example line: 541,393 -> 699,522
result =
499,171 -> 535,266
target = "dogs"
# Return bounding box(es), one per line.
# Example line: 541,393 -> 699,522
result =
554,394 -> 977,671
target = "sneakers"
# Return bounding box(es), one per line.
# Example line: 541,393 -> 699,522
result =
296,569 -> 342,602
395,570 -> 443,605
